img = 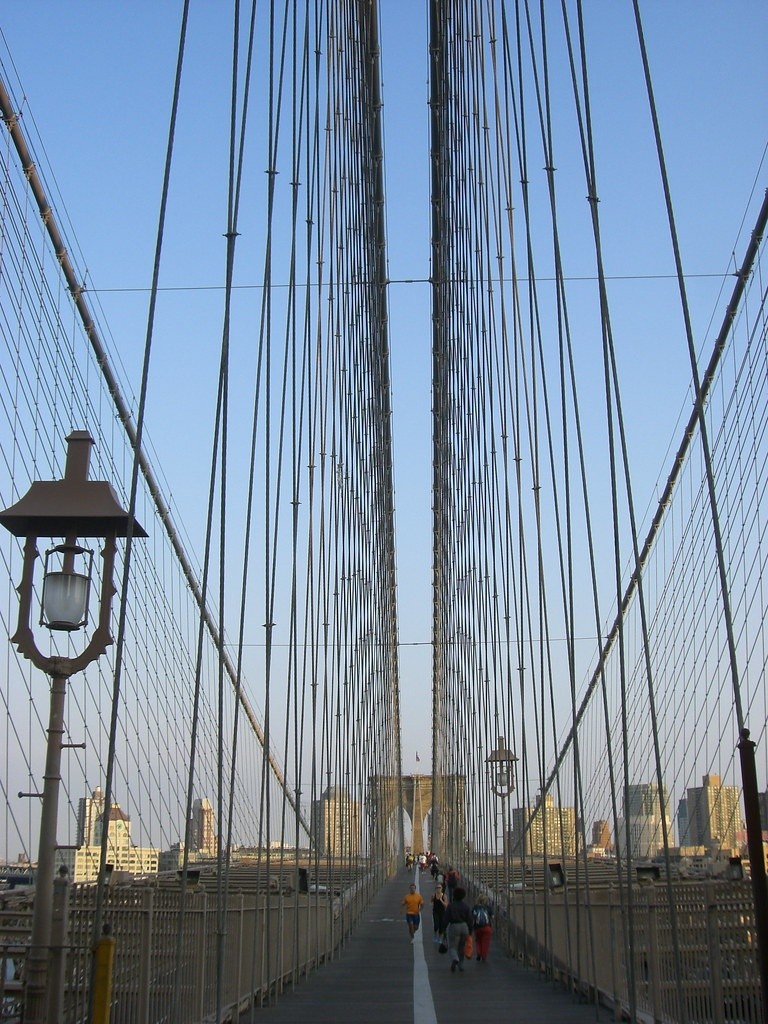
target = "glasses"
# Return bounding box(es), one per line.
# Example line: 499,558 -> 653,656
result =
435,887 -> 441,889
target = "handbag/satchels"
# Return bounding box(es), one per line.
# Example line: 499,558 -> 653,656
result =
466,935 -> 473,958
438,936 -> 448,954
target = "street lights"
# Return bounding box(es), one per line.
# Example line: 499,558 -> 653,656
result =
704,856 -> 744,1024
2,426 -> 149,1024
635,865 -> 662,1024
487,735 -> 521,948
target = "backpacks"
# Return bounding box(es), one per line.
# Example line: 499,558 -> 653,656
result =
476,909 -> 489,927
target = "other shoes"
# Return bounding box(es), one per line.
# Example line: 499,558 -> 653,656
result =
433,935 -> 442,944
451,958 -> 459,971
410,938 -> 414,944
476,957 -> 480,961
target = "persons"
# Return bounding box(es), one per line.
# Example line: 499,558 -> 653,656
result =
402,851 -> 495,972
